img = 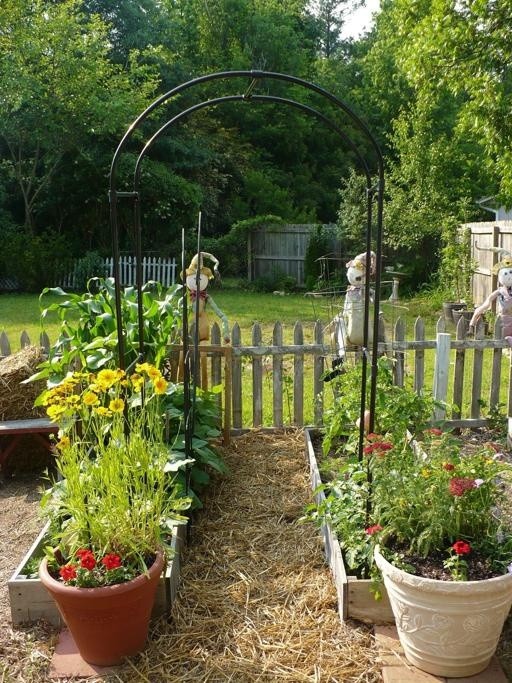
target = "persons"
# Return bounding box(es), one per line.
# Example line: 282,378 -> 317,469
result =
176,250 -> 230,392
462,240 -> 512,349
330,250 -> 381,349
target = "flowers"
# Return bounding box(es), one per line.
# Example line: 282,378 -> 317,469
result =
343,425 -> 511,574
29,359 -> 173,579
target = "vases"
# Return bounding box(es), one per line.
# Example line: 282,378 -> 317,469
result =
370,526 -> 512,680
34,542 -> 165,668
